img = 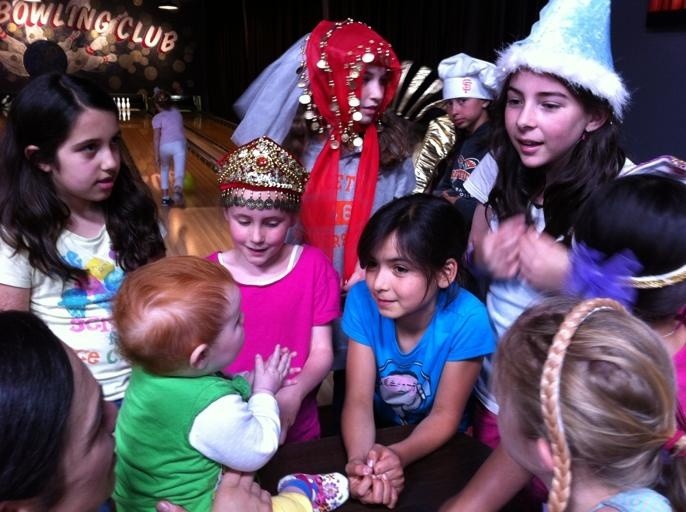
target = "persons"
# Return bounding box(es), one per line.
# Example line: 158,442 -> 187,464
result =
0,16 -> 686,512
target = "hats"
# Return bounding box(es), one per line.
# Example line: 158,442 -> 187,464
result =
437,53 -> 496,101
496,0 -> 633,124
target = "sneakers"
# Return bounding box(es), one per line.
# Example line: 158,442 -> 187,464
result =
277,472 -> 350,512
160,192 -> 186,207
